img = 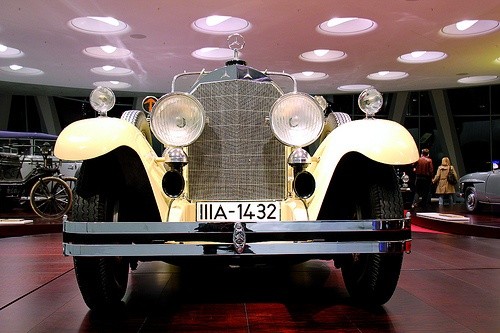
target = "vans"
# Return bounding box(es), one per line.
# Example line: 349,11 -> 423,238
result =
0,130 -> 83,221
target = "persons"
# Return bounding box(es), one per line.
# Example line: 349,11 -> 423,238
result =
412,149 -> 434,212
432,158 -> 457,213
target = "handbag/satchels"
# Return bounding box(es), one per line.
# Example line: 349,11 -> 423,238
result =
447,166 -> 457,185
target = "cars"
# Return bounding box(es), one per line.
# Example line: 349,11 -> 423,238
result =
53,32 -> 420,311
457,160 -> 500,214
